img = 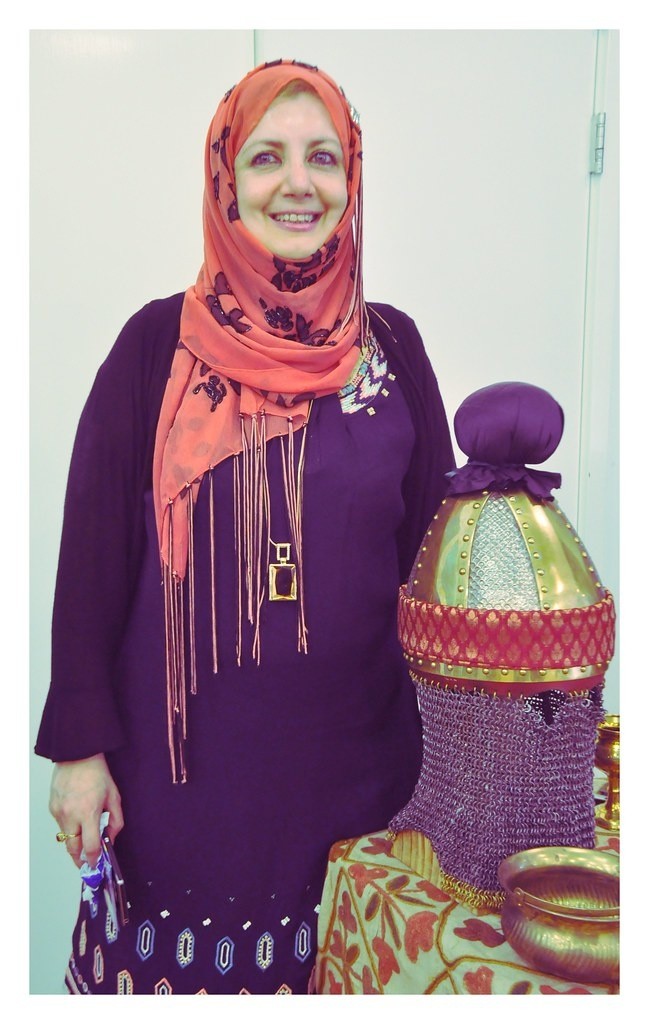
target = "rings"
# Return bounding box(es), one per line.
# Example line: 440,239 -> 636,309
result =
53,833 -> 82,841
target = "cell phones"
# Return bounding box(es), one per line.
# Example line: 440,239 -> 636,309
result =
102,834 -> 129,928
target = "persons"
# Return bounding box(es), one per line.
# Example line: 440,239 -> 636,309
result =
34,57 -> 456,995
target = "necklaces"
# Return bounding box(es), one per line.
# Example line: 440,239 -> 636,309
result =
251,399 -> 313,603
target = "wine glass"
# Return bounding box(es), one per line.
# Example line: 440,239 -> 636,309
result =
595,729 -> 620,831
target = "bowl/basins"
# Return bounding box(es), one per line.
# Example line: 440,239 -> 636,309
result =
498,846 -> 620,984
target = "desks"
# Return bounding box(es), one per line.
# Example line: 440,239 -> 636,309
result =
313,822 -> 620,995
